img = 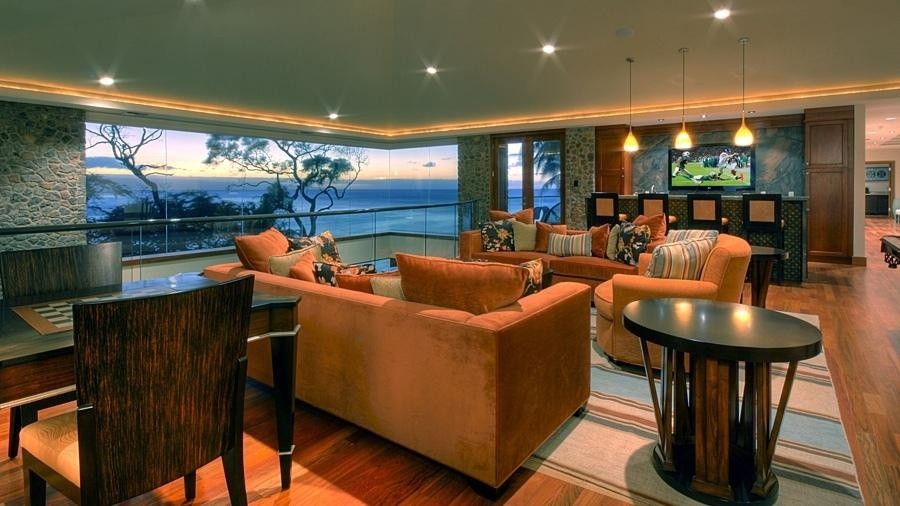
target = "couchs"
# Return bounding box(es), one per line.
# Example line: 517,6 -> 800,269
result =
458,224 -> 666,304
204,259 -> 591,501
595,233 -> 750,379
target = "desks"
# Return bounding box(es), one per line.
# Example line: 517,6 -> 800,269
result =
749,246 -> 789,309
621,298 -> 823,506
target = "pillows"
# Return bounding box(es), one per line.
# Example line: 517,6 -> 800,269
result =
334,273 -> 401,295
546,232 -> 592,257
661,229 -> 720,244
394,252 -> 529,315
479,219 -> 515,252
512,258 -> 545,299
587,224 -> 610,260
533,221 -> 569,253
511,220 -> 539,253
234,228 -> 287,276
614,220 -> 651,266
647,238 -> 717,282
317,262 -> 375,287
487,209 -> 532,226
631,214 -> 666,240
313,260 -> 375,290
268,245 -> 326,278
290,252 -> 322,286
288,231 -> 341,264
369,276 -> 406,302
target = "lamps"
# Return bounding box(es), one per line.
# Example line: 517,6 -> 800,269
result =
623,57 -> 639,152
735,37 -> 755,147
675,48 -> 693,149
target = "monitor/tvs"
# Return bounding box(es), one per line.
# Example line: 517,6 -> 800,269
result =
667,143 -> 756,192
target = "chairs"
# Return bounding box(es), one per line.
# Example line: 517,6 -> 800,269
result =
635,194 -> 678,233
18,274 -> 254,506
740,194 -> 786,286
0,240 -> 124,459
589,192 -> 627,230
687,193 -> 730,233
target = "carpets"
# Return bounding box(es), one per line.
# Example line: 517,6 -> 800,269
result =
513,309 -> 865,506
